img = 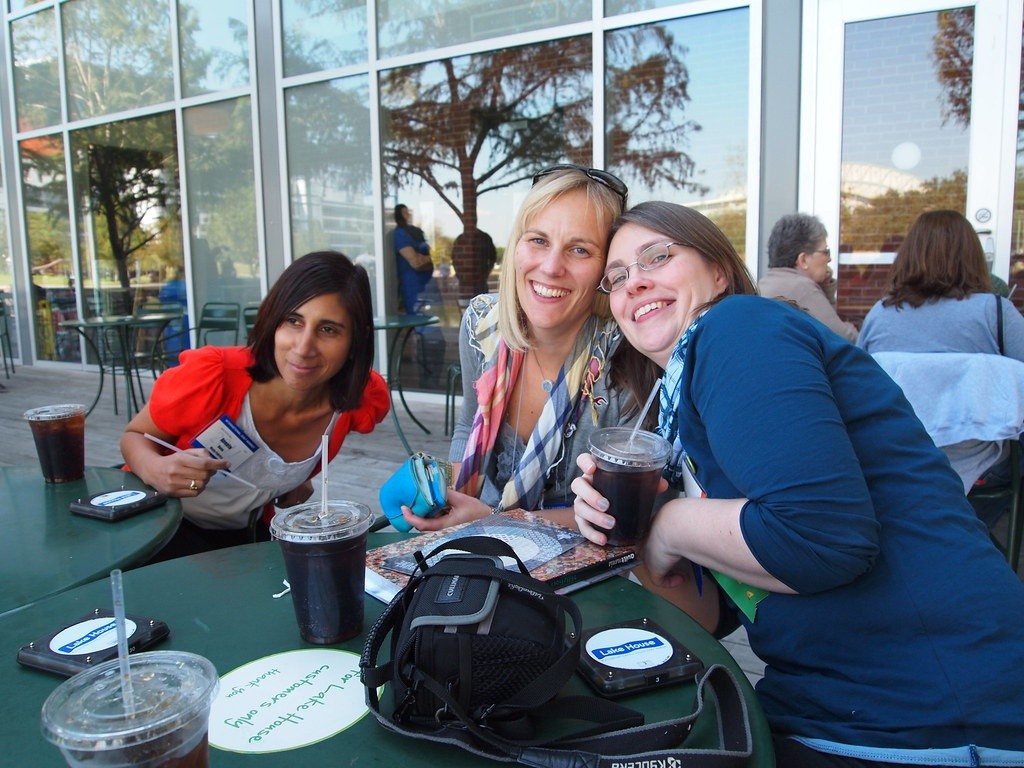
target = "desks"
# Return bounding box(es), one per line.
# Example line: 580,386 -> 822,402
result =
0,466 -> 183,614
373,315 -> 440,456
0,532 -> 776,768
57,313 -> 183,423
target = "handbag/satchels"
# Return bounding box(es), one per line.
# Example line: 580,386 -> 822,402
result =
360,537 -> 753,768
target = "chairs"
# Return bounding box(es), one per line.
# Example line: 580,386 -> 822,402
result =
0,272 -> 1024,572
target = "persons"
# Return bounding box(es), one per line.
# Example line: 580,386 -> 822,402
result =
761,214 -> 859,343
858,235 -> 905,302
114,251 -> 393,567
571,202 -> 1024,767
399,170 -> 644,531
853,210 -> 1024,528
452,212 -> 496,299
390,203 -> 447,363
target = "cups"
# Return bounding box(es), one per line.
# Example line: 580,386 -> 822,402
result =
23,403 -> 89,485
270,500 -> 376,644
587,427 -> 673,545
40,650 -> 220,768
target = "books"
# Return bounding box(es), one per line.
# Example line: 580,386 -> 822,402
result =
364,508 -> 641,594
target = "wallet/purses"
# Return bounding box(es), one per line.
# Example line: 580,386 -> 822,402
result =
379,454 -> 449,536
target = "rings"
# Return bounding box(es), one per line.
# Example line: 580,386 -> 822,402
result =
191,480 -> 197,490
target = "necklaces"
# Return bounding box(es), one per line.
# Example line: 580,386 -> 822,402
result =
531,343 -> 553,393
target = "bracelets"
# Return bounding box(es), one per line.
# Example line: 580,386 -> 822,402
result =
490,505 -> 500,515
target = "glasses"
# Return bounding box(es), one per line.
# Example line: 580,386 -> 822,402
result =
813,246 -> 831,259
532,163 -> 629,216
594,241 -> 695,295
